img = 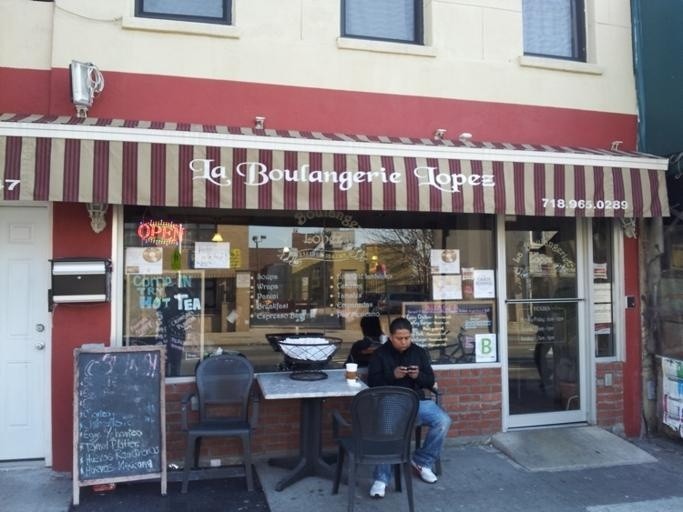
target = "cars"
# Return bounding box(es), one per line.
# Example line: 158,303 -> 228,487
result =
378,293 -> 429,314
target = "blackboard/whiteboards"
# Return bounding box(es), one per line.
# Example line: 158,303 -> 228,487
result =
401,300 -> 496,365
72,345 -> 166,487
126,270 -> 205,383
516,306 -> 567,344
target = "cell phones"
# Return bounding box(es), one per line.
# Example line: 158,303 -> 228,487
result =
407,365 -> 418,370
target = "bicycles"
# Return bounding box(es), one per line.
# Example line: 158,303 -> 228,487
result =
423,327 -> 475,364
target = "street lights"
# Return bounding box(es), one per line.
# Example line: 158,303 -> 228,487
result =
252,234 -> 267,292
362,244 -> 378,295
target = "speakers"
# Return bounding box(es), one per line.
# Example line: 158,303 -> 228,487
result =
71,60 -> 92,104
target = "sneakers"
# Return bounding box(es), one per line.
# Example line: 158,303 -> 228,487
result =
369,480 -> 386,498
411,460 -> 437,484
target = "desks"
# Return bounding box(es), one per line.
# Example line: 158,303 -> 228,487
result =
256,370 -> 369,491
331,386 -> 419,512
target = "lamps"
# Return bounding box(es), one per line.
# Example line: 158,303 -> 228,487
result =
459,133 -> 472,140
619,217 -> 637,240
434,129 -> 447,139
86,202 -> 108,234
610,141 -> 623,151
255,116 -> 265,130
212,233 -> 223,242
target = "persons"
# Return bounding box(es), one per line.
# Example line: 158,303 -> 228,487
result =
342,314 -> 387,366
463,283 -> 474,300
530,279 -> 557,387
368,316 -> 452,498
154,284 -> 187,376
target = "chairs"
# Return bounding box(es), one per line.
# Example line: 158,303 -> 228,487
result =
415,386 -> 445,474
180,354 -> 260,494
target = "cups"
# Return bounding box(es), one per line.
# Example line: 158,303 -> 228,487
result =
346,363 -> 358,385
